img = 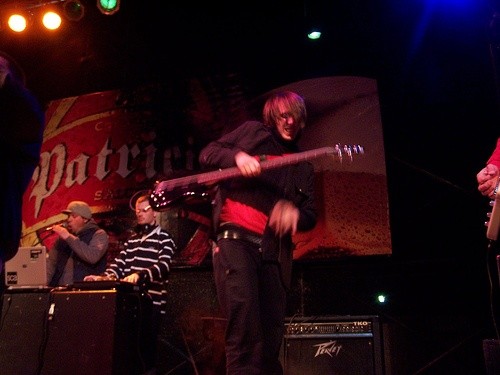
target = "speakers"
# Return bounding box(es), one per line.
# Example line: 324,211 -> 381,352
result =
285,315 -> 380,375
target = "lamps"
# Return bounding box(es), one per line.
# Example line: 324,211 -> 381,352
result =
63,0 -> 85,21
95,0 -> 120,16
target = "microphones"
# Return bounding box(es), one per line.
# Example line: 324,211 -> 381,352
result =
45,222 -> 68,230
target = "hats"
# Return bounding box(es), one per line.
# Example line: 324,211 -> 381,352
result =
61,200 -> 92,219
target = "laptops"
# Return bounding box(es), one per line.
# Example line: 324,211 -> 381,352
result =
5,246 -> 52,291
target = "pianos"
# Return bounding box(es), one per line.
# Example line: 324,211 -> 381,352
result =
70,279 -> 142,298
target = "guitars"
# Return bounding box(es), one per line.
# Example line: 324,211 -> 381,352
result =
149,142 -> 368,212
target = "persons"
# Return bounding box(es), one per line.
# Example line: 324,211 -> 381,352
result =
0,199 -> 19,313
84,196 -> 178,368
476,137 -> 500,199
46,201 -> 108,287
199,89 -> 317,375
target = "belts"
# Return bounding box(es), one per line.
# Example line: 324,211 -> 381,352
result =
215,230 -> 264,245
